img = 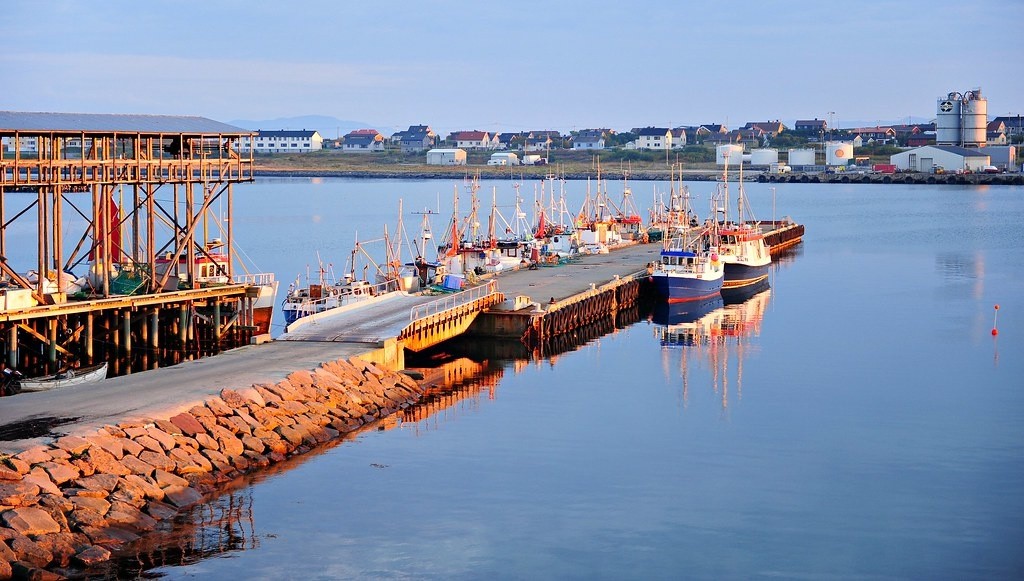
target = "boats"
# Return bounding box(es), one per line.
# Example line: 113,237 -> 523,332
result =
708,150 -> 772,289
283,153 -> 723,326
